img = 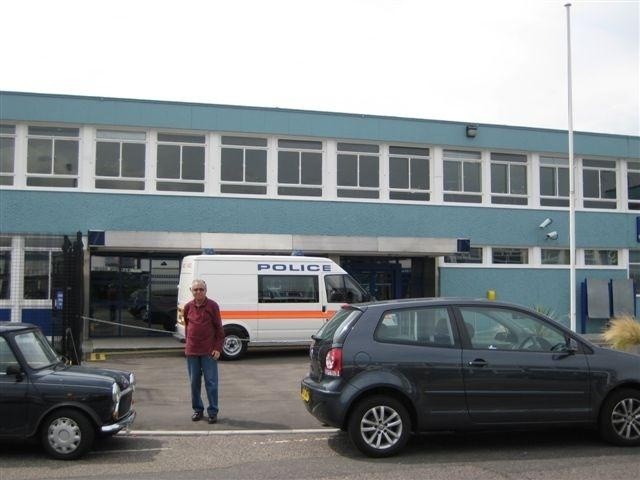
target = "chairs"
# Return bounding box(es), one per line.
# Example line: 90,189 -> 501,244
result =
434,319 -> 474,346
378,323 -> 390,340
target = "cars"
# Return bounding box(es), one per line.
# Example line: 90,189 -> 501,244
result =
301,296 -> 639,458
0,321 -> 136,461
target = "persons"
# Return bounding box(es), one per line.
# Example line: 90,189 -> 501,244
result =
183,280 -> 225,424
99,283 -> 129,322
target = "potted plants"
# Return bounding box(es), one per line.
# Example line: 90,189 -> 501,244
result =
605,312 -> 640,355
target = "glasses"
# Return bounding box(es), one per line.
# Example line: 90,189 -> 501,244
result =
193,288 -> 205,291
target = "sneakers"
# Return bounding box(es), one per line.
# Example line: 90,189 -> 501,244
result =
208,415 -> 217,423
191,412 -> 203,421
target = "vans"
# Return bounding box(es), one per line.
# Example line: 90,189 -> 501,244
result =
174,254 -> 398,360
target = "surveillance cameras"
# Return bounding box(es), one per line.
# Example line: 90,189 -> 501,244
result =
546,231 -> 557,238
539,217 -> 552,229
